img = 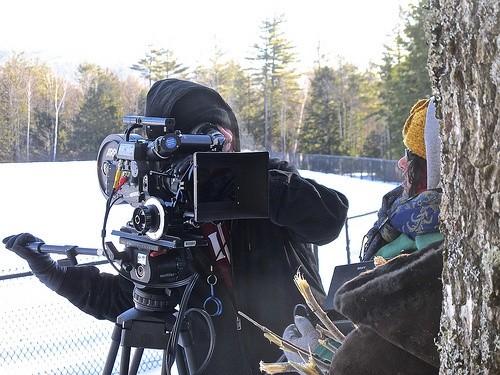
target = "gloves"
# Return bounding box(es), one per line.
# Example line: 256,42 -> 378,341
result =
282,304 -> 324,374
172,152 -> 196,177
3,232 -> 67,293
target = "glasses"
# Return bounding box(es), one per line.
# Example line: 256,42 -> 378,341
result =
404,148 -> 410,162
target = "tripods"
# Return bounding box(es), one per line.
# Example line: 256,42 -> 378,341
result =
24,242 -> 198,375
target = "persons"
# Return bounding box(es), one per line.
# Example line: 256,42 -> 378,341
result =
1,78 -> 350,375
279,99 -> 440,375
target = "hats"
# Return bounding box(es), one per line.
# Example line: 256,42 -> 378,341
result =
402,97 -> 434,162
169,90 -> 232,137
